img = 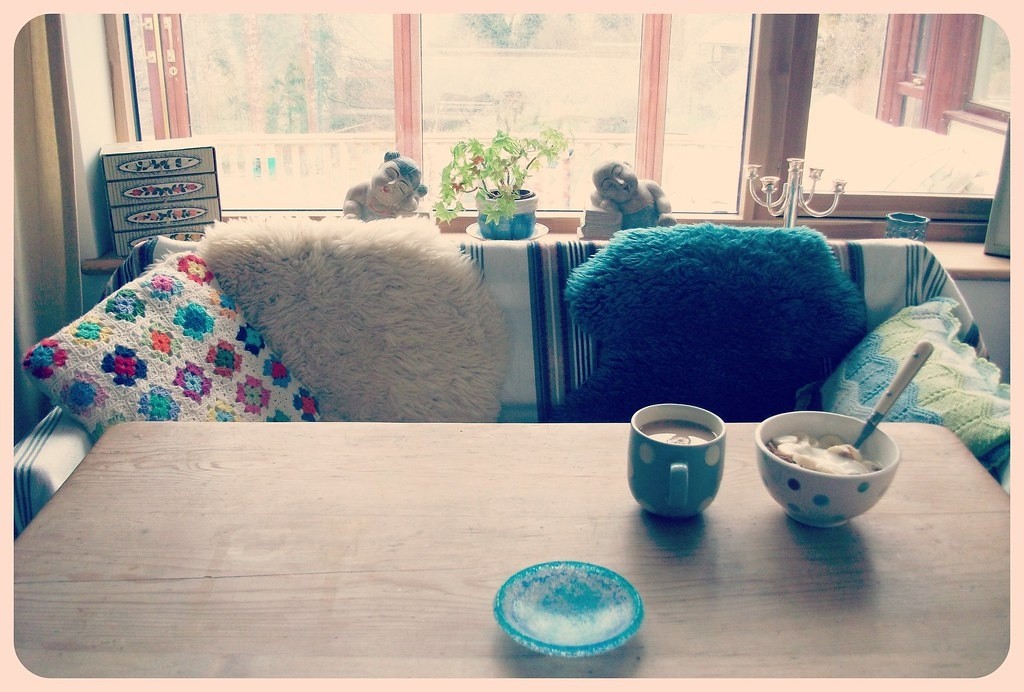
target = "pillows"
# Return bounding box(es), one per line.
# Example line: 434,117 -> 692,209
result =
23,252 -> 322,438
815,290 -> 1011,481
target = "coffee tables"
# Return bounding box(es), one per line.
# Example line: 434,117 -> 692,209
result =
11,419 -> 1013,679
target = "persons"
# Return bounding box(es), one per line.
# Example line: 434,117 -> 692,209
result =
591,159 -> 679,230
342,150 -> 429,218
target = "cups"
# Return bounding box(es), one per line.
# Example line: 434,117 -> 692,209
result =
884,210 -> 930,244
628,402 -> 729,520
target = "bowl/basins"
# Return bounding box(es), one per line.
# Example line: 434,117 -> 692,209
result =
756,411 -> 900,529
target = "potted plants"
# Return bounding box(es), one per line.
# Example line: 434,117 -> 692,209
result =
434,127 -> 572,243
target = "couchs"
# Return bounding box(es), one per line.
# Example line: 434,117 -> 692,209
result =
14,224 -> 1010,541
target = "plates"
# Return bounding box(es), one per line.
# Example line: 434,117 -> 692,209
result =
493,561 -> 644,660
465,222 -> 548,242
